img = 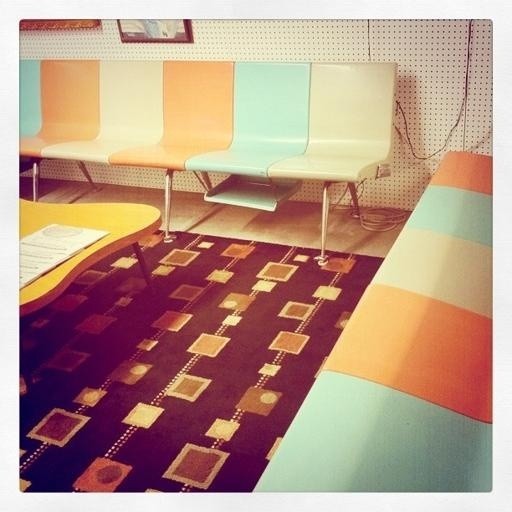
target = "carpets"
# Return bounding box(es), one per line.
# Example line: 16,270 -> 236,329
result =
20,229 -> 386,493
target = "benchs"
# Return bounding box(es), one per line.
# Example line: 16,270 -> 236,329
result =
251,152 -> 492,491
20,59 -> 398,264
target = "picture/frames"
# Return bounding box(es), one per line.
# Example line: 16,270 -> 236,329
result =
117,19 -> 195,43
